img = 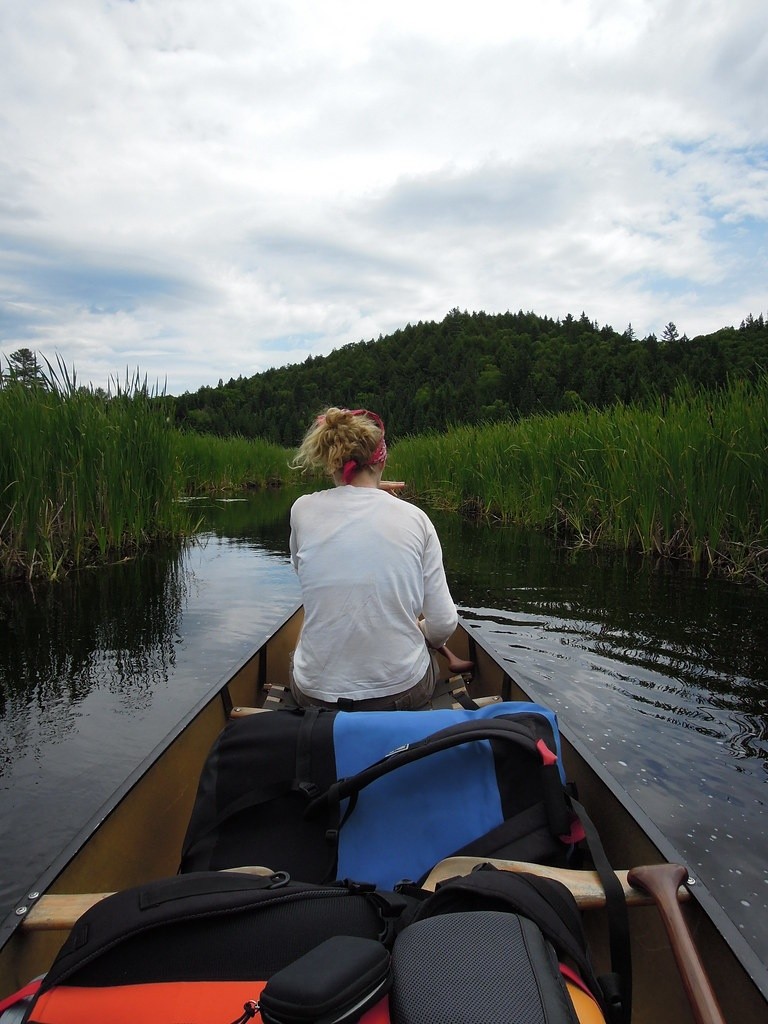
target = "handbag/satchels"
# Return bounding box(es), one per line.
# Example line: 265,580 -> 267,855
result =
386,862 -> 610,1024
176,701 -> 578,895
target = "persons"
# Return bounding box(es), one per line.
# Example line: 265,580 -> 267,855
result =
290,408 -> 462,712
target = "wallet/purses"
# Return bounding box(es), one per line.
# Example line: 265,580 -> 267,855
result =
259,936 -> 391,1024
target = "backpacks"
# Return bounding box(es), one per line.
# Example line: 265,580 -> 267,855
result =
21,872 -> 394,1024
